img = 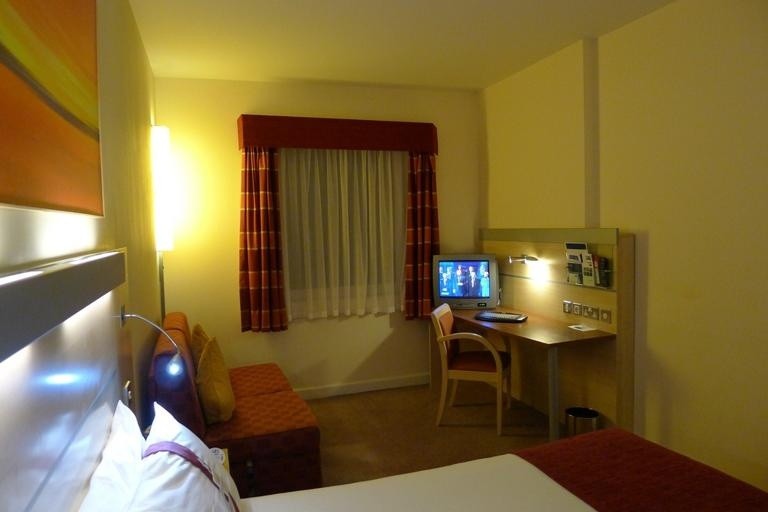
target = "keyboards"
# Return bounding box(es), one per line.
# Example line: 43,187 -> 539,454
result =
474,310 -> 529,323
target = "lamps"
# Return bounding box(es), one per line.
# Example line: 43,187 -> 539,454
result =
507,255 -> 539,264
146,121 -> 178,320
118,304 -> 186,377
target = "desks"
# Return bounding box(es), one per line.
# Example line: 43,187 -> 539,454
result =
445,307 -> 617,442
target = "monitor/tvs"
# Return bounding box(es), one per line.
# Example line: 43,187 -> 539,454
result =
432,254 -> 500,309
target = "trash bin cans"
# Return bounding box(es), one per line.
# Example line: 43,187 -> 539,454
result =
564,407 -> 599,438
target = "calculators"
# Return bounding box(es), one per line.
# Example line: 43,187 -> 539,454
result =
565,242 -> 587,259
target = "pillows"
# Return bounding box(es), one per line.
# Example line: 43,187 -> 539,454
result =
80,394 -> 146,512
191,321 -> 209,366
136,396 -> 239,511
194,338 -> 236,423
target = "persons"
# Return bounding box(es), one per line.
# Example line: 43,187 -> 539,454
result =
439,264 -> 489,297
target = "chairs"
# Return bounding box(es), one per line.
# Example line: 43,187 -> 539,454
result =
146,328 -> 323,499
428,303 -> 513,433
160,311 -> 292,397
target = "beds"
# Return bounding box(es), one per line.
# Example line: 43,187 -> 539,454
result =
0,244 -> 767,512
